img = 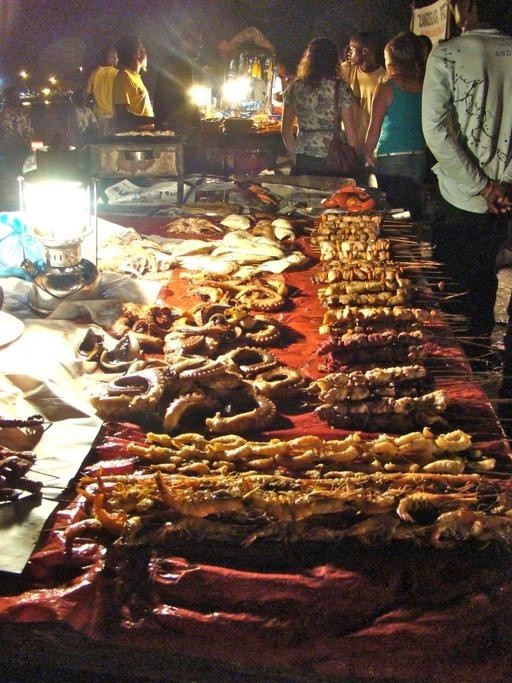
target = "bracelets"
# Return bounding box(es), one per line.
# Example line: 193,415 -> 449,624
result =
482,178 -> 494,199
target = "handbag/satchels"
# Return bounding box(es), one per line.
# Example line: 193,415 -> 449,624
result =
322,135 -> 360,176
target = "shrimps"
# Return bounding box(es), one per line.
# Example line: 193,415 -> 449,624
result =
66,468 -> 512,568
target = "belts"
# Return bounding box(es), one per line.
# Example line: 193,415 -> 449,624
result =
374,150 -> 426,157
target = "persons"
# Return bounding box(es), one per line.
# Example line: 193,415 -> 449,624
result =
1,24 -> 439,218
421,0 -> 512,370
